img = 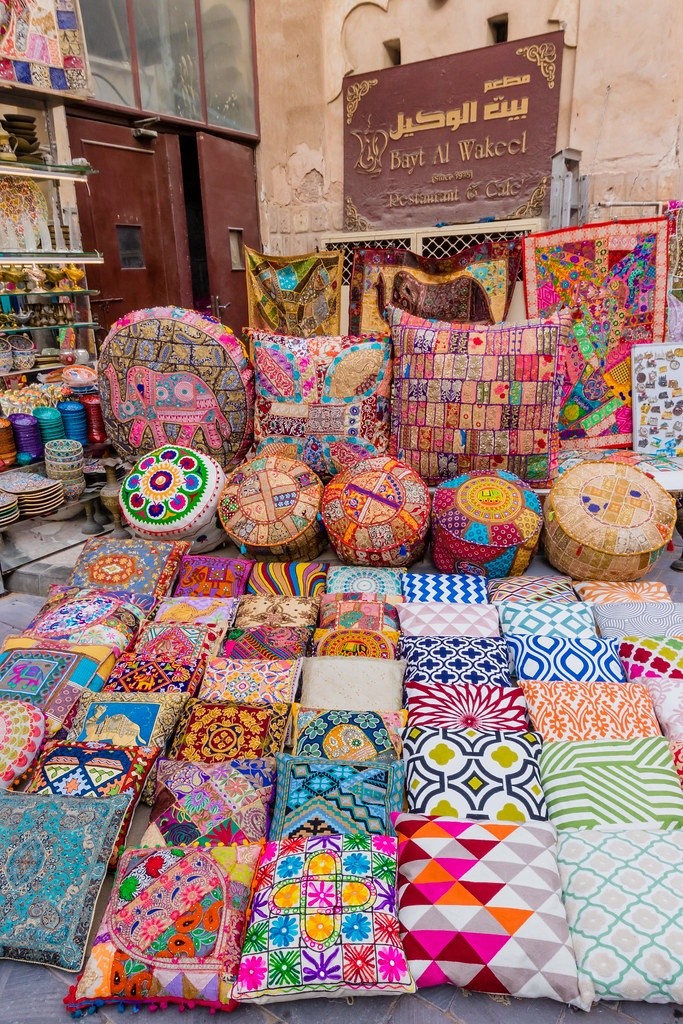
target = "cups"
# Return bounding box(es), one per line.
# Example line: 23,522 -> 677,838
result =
41,347 -> 60,355
73,348 -> 90,363
58,348 -> 77,365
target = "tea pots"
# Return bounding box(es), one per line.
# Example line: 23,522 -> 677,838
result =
0,264 -> 85,292
0,302 -> 56,329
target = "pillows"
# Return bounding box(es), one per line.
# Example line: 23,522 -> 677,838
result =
98,303 -> 572,489
1,537 -> 683,1019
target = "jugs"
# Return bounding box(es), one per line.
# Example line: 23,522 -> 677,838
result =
0,129 -> 19,162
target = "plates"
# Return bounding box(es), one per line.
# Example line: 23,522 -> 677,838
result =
0,470 -> 66,527
62,364 -> 100,395
35,354 -> 60,363
83,458 -> 126,493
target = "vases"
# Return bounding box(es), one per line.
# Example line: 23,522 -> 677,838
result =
99,457 -> 132,540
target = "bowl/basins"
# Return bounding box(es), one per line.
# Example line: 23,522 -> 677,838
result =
45,439 -> 87,503
0,395 -> 109,472
0,335 -> 36,374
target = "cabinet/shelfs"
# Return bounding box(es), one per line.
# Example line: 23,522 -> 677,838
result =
0,160 -> 104,376
